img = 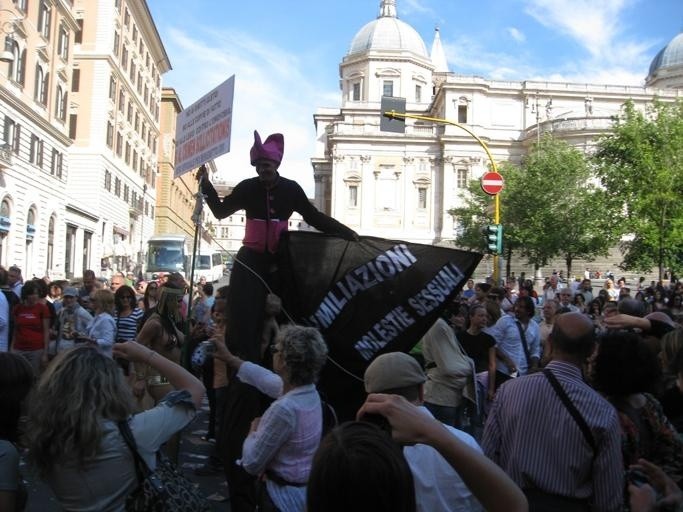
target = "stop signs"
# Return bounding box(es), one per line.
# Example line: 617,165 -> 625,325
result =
482,172 -> 503,194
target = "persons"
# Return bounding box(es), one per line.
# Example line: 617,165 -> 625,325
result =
195,130 -> 361,385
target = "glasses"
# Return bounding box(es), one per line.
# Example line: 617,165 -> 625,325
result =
486,295 -> 499,300
120,296 -> 132,300
269,345 -> 282,354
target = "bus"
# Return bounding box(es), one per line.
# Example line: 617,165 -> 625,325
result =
145,236 -> 188,285
189,252 -> 222,283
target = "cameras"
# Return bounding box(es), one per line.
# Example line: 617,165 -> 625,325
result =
114,354 -> 129,376
202,341 -> 217,355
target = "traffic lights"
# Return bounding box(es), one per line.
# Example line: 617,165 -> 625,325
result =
486,225 -> 502,255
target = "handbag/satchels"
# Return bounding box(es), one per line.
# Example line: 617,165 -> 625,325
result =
126,463 -> 215,512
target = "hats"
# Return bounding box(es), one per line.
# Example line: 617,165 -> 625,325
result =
251,131 -> 285,165
364,350 -> 432,393
59,286 -> 79,298
